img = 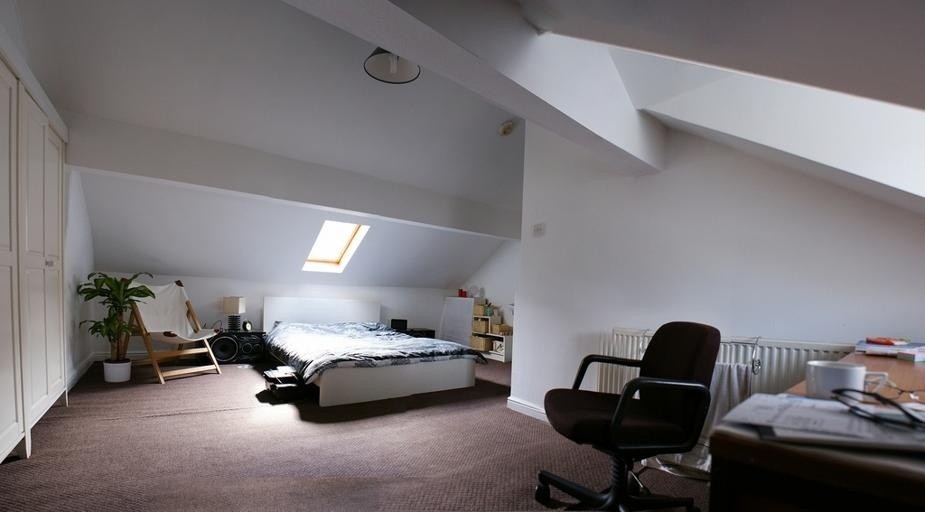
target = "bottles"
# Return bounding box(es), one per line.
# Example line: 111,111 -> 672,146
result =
458,288 -> 466,297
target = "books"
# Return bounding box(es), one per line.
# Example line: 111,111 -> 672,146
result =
854,338 -> 925,361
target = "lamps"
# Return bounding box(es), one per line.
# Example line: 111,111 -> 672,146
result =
220,296 -> 247,332
361,41 -> 421,85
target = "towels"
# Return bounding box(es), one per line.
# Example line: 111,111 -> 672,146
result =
667,363 -> 755,471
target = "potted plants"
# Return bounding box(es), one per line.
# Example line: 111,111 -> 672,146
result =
75,266 -> 162,383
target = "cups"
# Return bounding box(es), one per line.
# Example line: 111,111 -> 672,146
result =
806,360 -> 888,404
485,307 -> 492,316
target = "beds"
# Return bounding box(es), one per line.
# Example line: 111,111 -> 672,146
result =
261,294 -> 480,408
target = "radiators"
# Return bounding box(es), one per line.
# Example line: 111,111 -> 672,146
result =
598,325 -> 854,473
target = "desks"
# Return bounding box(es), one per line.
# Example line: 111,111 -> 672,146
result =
715,342 -> 925,512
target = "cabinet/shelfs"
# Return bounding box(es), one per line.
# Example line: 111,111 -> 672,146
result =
471,315 -> 513,364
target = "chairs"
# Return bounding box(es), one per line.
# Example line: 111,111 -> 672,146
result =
532,320 -> 720,509
122,279 -> 222,382
